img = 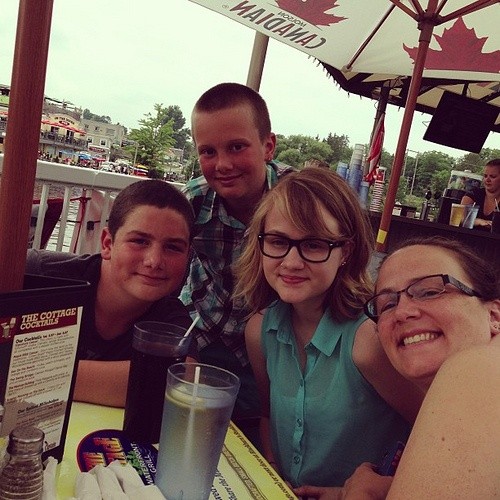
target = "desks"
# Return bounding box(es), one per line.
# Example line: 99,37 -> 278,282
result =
0,399 -> 298,500
365,209 -> 500,289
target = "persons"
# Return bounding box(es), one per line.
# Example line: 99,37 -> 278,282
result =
23,179 -> 201,410
180,82 -> 302,459
37,130 -> 132,174
363,159 -> 500,500
235,165 -> 431,500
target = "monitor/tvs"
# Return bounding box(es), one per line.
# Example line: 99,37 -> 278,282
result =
423,90 -> 500,154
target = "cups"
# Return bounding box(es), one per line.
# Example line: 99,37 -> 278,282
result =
420,201 -> 431,221
461,205 -> 479,229
122,321 -> 193,444
154,362 -> 240,500
335,144 -> 387,212
449,203 -> 465,227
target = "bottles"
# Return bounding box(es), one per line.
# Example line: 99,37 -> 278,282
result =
0,425 -> 45,500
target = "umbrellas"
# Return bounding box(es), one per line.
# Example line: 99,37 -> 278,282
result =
192,0 -> 500,255
92,156 -> 105,162
41,120 -> 85,139
60,150 -> 73,158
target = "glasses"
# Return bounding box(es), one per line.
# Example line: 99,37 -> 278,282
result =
258,228 -> 346,262
364,274 -> 482,324
